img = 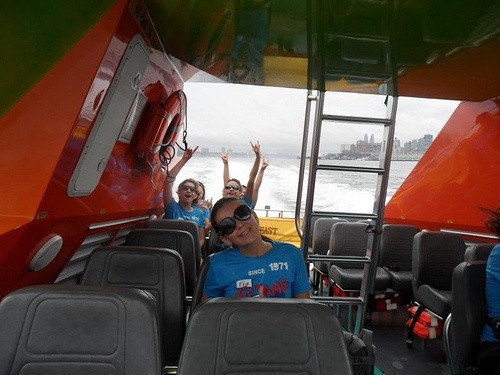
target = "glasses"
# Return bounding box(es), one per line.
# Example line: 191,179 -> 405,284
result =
183,186 -> 195,191
218,205 -> 252,235
225,185 -> 239,190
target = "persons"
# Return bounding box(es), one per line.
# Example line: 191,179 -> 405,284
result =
217,149 -> 270,210
201,196 -> 312,303
194,180 -> 213,230
163,145 -> 206,266
477,204 -> 500,375
222,140 -> 261,210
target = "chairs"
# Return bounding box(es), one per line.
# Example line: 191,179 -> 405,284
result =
0,217 -> 500,375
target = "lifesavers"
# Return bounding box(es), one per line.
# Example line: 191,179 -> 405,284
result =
135,90 -> 188,175
